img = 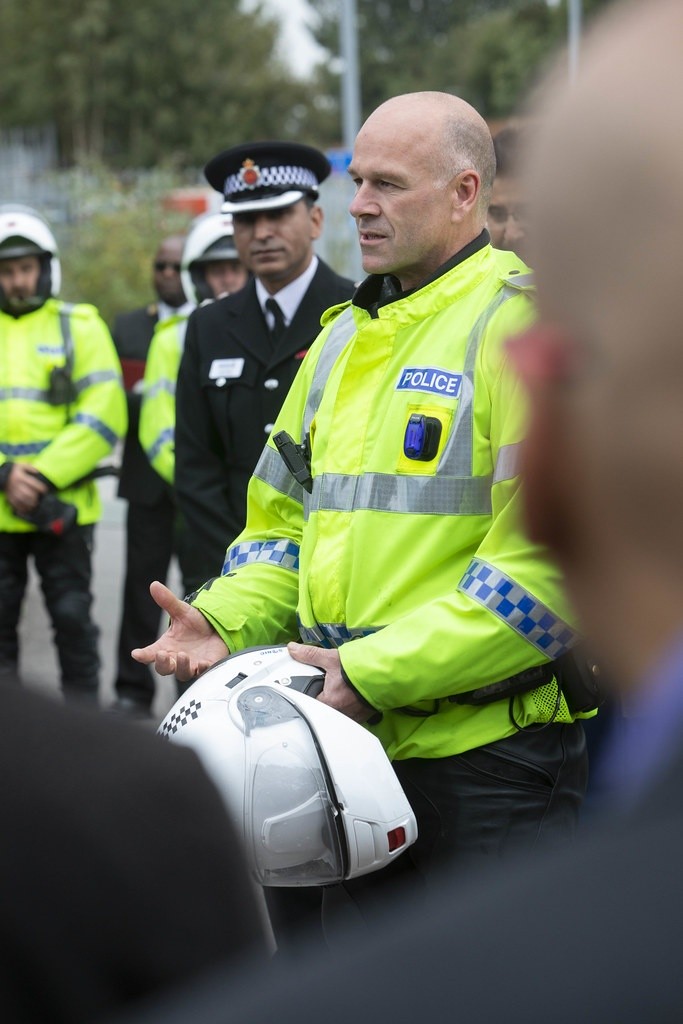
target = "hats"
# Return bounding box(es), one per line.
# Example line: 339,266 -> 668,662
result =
202,143 -> 334,214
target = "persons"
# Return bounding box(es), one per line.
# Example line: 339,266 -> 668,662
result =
114,91 -> 610,947
0,202 -> 127,708
0,676 -> 268,1024
134,0 -> 683,1024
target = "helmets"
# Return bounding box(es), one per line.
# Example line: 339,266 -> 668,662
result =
180,211 -> 234,304
1,200 -> 63,298
150,644 -> 420,886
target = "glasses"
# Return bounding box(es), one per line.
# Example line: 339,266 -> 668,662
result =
150,260 -> 183,274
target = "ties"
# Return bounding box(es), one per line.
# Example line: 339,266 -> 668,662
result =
264,298 -> 289,337
